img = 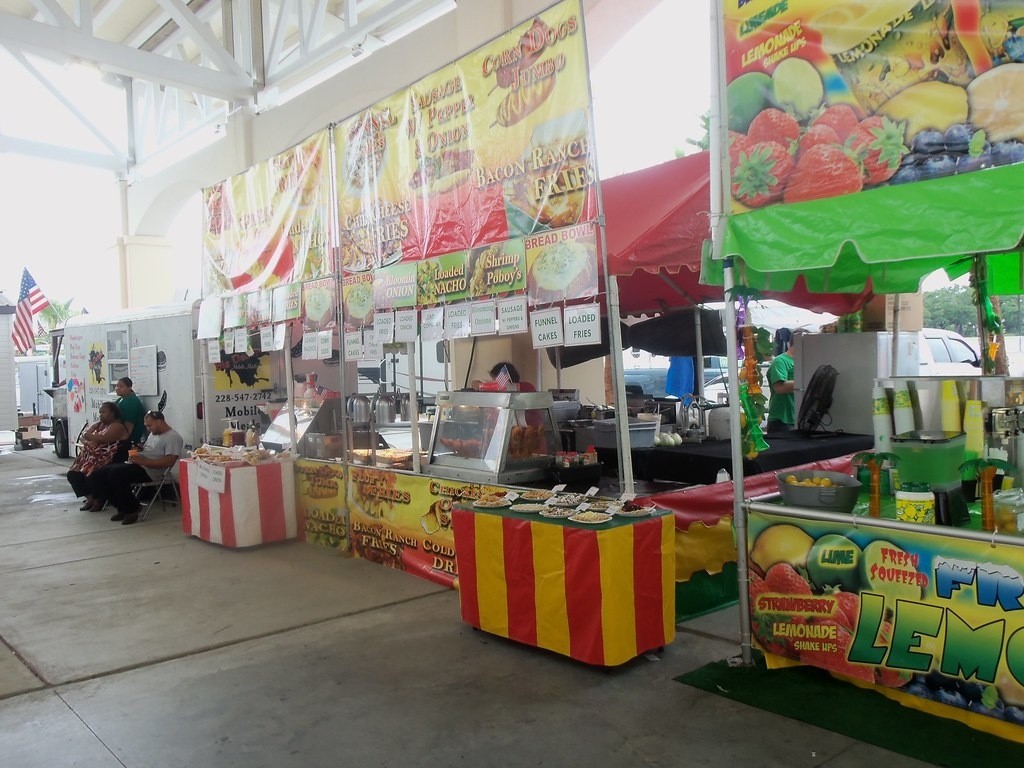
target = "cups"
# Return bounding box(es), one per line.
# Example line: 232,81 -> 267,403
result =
941,380 -> 984,460
128,450 -> 137,461
872,378 -> 915,452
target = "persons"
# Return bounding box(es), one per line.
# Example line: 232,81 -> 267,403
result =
767,328 -> 811,434
67,377 -> 183,525
472,362 -> 548,455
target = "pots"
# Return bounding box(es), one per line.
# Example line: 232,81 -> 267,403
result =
553,397 -> 580,420
304,433 -> 343,459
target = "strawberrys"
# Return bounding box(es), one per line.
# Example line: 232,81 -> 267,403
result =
745,562 -> 912,688
725,103 -> 909,206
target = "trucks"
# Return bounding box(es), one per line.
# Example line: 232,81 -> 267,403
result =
43,299 -> 358,484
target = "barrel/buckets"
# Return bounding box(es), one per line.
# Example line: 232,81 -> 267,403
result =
776,470 -> 862,513
992,407 -> 1018,437
637,404 -> 661,435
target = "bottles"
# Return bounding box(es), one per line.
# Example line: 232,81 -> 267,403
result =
992,488 -> 1024,535
894,481 -> 934,525
245,419 -> 258,446
555,445 -> 597,467
654,426 -> 689,447
347,392 -> 419,423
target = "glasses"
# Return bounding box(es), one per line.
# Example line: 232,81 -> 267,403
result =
147,410 -> 161,418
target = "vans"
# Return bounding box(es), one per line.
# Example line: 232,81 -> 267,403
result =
918,328 -> 982,377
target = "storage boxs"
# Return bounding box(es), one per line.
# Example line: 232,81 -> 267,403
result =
862,291 -> 923,330
303,433 -> 343,460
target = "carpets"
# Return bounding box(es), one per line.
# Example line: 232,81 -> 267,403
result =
671,650 -> 1024,768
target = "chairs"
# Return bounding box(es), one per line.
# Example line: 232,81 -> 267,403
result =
102,441 -> 180,522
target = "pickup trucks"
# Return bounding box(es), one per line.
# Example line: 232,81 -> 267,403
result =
623,357 -> 744,417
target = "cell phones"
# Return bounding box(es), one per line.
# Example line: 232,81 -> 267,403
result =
131,441 -> 143,452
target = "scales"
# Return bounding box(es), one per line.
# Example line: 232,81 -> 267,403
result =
545,389 -> 581,423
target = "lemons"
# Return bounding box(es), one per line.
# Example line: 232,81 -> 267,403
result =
785,475 -> 840,486
868,82 -> 968,146
751,524 -> 923,602
727,56 -> 823,133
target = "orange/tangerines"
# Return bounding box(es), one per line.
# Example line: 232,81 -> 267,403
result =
962,60 -> 1024,147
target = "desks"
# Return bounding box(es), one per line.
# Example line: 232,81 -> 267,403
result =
179,457 -> 298,551
596,430 -> 876,486
451,492 -> 675,674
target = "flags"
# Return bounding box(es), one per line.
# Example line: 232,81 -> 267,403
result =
12,268 -> 50,354
496,366 -> 510,388
37,321 -> 46,336
314,386 -> 329,400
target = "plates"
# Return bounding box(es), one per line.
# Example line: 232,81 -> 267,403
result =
473,492 -> 656,524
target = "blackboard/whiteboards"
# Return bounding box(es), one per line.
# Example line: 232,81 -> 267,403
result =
130,344 -> 158,397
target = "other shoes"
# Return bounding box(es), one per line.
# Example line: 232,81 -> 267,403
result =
111,512 -> 124,521
90,499 -> 106,512
122,504 -> 143,525
80,502 -> 92,510
